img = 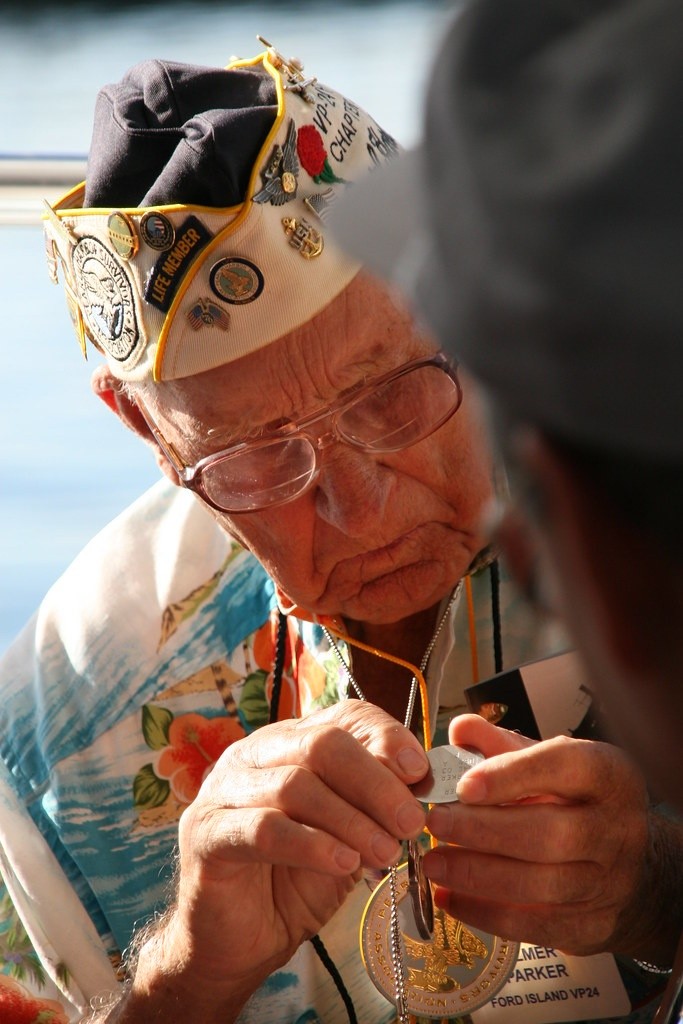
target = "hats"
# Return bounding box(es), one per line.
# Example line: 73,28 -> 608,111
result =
36,33 -> 419,385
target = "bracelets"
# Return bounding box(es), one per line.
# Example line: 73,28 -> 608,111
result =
630,955 -> 675,974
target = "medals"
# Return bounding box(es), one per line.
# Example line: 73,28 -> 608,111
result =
358,853 -> 518,1018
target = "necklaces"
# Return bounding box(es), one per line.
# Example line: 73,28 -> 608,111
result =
303,576 -> 470,1021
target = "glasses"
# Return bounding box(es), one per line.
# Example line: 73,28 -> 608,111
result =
177,346 -> 465,516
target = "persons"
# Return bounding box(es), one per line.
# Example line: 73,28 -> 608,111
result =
325,2 -> 682,1024
1,29 -> 679,1023
